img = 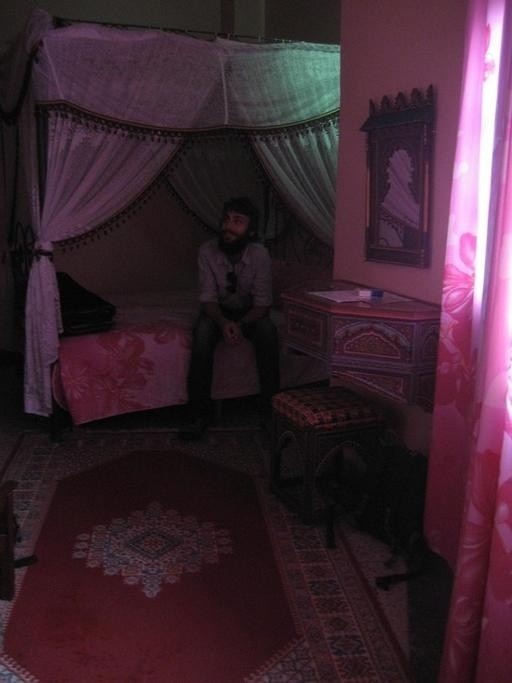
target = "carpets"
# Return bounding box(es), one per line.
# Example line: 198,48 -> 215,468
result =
0,424 -> 408,683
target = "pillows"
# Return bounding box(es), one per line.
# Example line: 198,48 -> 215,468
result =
270,256 -> 330,307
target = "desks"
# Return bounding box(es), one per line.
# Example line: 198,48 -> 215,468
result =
280,279 -> 441,405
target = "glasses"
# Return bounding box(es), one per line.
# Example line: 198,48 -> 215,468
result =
226,272 -> 238,295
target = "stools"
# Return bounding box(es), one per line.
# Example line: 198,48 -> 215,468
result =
270,386 -> 397,525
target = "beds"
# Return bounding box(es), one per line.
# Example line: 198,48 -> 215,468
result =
0,7 -> 341,442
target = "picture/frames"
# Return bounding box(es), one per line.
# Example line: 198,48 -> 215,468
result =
359,84 -> 434,268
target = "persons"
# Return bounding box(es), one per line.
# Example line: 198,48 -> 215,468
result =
180,198 -> 281,440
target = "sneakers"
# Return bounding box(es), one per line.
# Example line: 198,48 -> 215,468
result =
178,410 -> 213,442
